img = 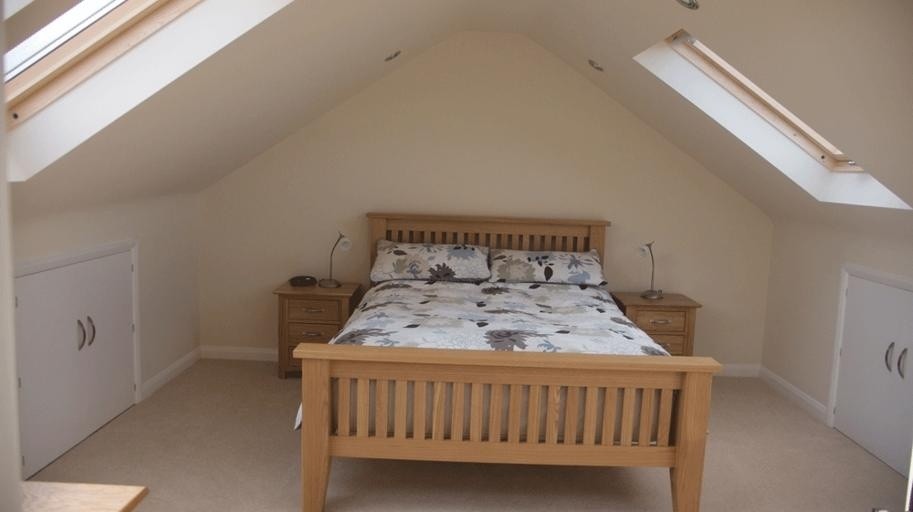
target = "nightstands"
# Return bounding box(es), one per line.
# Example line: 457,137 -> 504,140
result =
611,290 -> 704,358
272,278 -> 361,379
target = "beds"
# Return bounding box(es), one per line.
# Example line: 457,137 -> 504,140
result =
289,210 -> 723,512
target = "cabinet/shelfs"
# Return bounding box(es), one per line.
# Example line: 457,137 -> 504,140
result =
834,276 -> 911,478
12,248 -> 139,480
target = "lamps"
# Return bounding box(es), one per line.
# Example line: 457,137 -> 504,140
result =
319,232 -> 353,288
638,242 -> 665,300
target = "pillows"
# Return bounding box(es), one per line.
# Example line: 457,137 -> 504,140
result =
370,239 -> 608,287
